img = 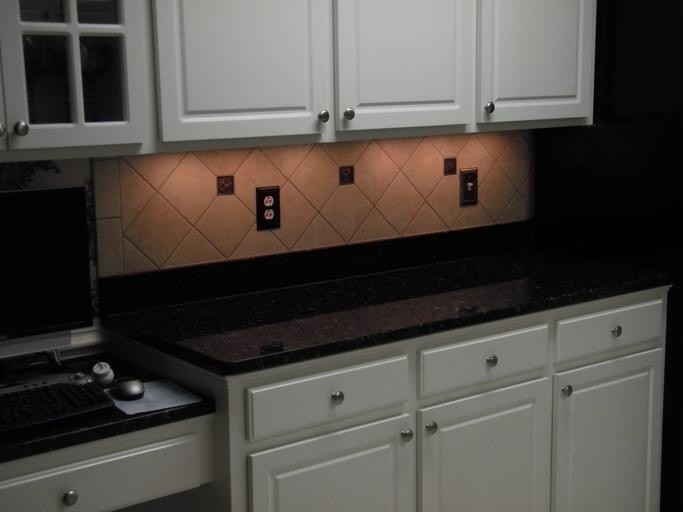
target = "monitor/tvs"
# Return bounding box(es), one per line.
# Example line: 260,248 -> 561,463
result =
0,183 -> 103,361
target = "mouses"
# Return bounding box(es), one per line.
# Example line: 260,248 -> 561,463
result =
111,377 -> 145,400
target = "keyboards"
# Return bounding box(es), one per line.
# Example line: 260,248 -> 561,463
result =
0,373 -> 115,431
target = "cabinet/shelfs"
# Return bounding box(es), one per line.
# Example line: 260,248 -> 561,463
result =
477,1 -> 597,133
0,0 -> 148,160
148,0 -> 476,153
552,281 -> 670,512
225,315 -> 552,512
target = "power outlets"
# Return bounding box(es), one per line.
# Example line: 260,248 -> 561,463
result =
256,185 -> 281,231
